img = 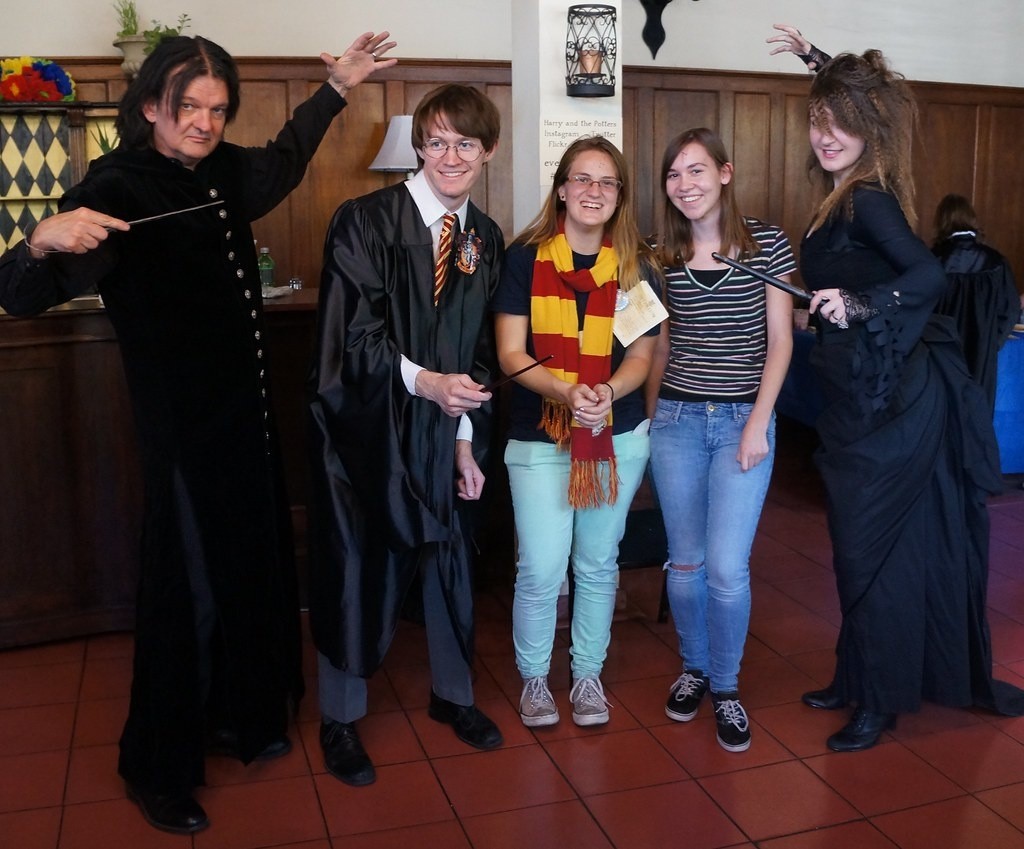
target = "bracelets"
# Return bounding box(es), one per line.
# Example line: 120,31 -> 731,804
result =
601,382 -> 614,402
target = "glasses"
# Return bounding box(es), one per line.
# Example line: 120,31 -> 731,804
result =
422,137 -> 484,161
567,176 -> 624,192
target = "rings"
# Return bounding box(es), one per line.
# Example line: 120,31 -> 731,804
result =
371,52 -> 377,58
838,319 -> 849,329
795,30 -> 803,36
831,313 -> 840,322
591,423 -> 604,437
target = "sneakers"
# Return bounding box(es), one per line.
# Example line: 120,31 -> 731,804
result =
569,678 -> 614,726
664,669 -> 709,721
711,693 -> 751,752
518,675 -> 559,726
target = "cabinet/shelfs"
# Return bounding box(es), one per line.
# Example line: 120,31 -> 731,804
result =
0,287 -> 329,645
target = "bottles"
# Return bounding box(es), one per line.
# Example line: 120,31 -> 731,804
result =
288,278 -> 303,290
258,248 -> 275,287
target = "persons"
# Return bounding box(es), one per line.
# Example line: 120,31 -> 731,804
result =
0,31 -> 397,832
310,83 -> 506,787
496,136 -> 661,727
766,24 -> 950,752
645,127 -> 796,752
928,194 -> 1021,507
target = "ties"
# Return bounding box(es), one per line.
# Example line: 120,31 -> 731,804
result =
435,214 -> 455,307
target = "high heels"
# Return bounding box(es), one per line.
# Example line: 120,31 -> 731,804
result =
802,677 -> 858,710
827,707 -> 897,751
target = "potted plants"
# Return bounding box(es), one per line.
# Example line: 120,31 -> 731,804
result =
111,0 -> 190,79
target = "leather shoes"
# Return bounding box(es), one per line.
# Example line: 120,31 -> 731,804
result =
319,716 -> 375,784
126,779 -> 208,833
204,724 -> 291,760
428,685 -> 501,747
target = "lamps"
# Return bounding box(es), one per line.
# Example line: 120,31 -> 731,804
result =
565,4 -> 616,97
369,116 -> 418,179
